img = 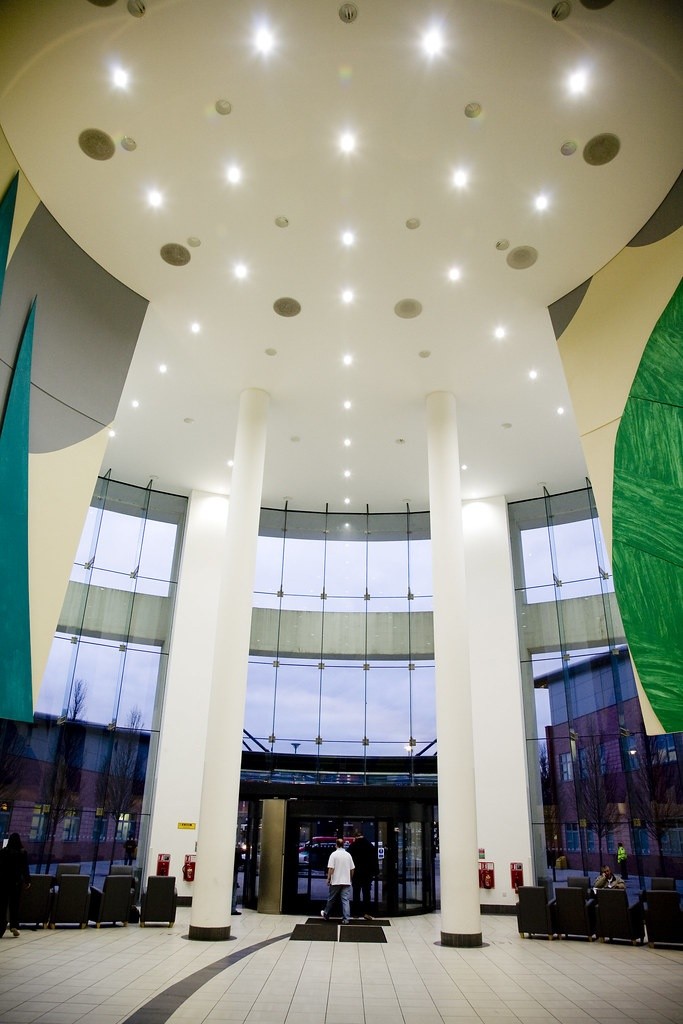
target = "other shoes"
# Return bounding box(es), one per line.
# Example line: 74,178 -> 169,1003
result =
342,918 -> 349,924
321,910 -> 329,920
604,937 -> 609,941
364,915 -> 372,920
354,913 -> 359,917
232,911 -> 242,915
592,933 -> 597,939
11,928 -> 19,936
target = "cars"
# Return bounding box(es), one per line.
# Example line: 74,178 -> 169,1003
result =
235,836 -> 358,872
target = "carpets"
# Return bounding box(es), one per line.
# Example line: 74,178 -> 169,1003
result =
290,923 -> 338,943
305,918 -> 390,926
338,926 -> 386,943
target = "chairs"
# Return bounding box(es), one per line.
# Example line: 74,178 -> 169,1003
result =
51,875 -> 90,930
639,878 -> 676,903
139,876 -> 178,927
55,864 -> 81,886
568,877 -> 592,897
88,876 -> 134,928
553,887 -> 596,941
640,891 -> 683,949
516,885 -> 556,940
11,874 -> 53,929
597,889 -> 644,946
110,865 -> 139,905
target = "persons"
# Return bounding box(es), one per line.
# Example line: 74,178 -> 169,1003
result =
0,832 -> 31,938
123,835 -> 136,866
232,848 -> 241,914
593,842 -> 630,892
320,829 -> 380,925
